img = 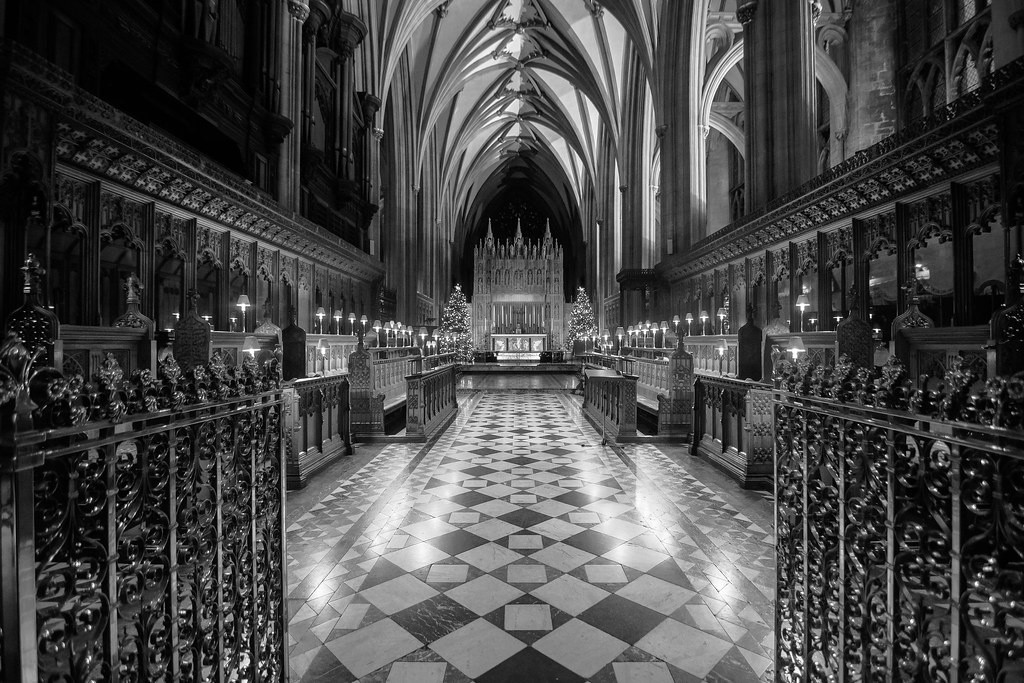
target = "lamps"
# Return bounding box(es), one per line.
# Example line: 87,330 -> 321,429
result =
673,315 -> 680,333
627,319 -> 659,348
795,294 -> 808,331
241,336 -> 261,359
432,329 -> 440,355
237,295 -> 250,333
373,320 -> 382,348
615,327 -> 626,349
419,327 -> 430,350
333,310 -> 343,335
787,337 -> 806,363
316,307 -> 325,334
348,313 -> 356,336
700,311 -> 710,336
383,321 -> 414,349
685,313 -> 694,336
717,309 -> 727,335
361,315 -> 368,337
660,321 -> 669,348
